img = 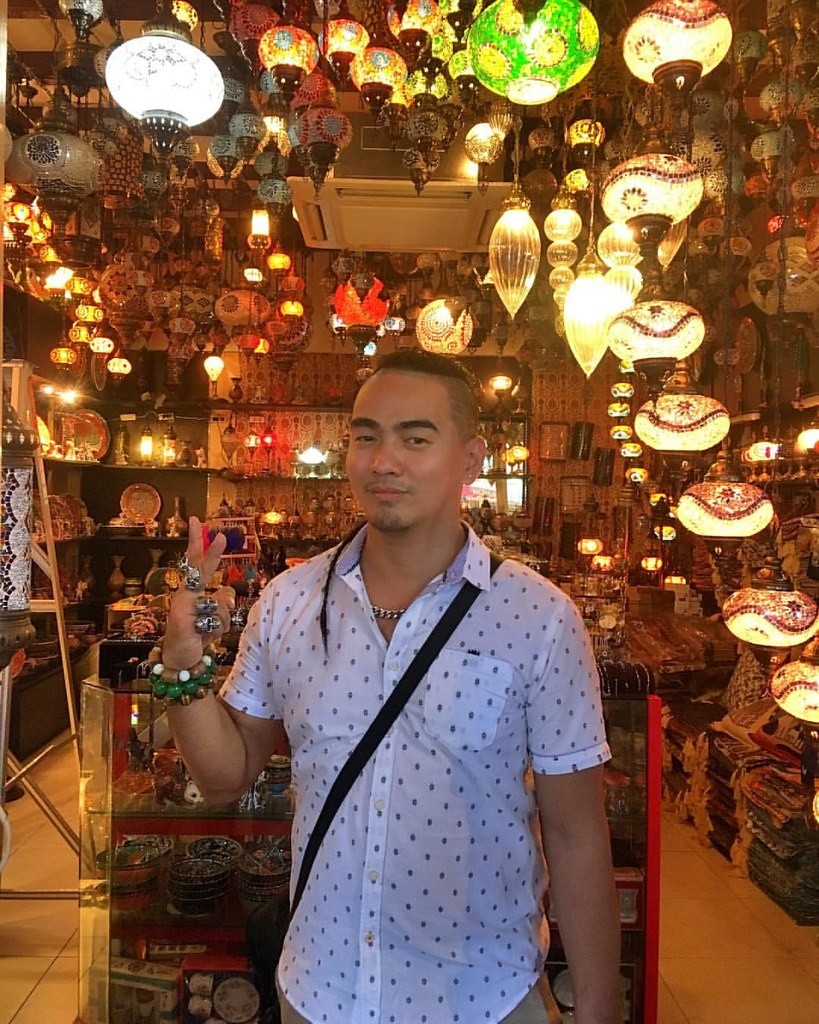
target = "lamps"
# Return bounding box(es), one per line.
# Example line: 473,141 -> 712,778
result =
1,0 -> 818,399
721,546 -> 819,686
634,355 -> 729,478
771,630 -> 819,765
736,417 -> 819,482
674,437 -> 774,574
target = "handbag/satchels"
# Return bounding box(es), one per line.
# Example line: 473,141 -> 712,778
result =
247,899 -> 290,997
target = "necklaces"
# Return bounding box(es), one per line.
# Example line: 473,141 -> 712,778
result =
370,603 -> 405,621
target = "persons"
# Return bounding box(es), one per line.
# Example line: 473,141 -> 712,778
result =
147,348 -> 631,1024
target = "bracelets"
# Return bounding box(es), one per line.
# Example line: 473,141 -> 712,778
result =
147,634 -> 219,684
146,659 -> 218,698
152,674 -> 216,706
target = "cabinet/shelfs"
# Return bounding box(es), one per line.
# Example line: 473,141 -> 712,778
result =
2,399 -> 660,1023
